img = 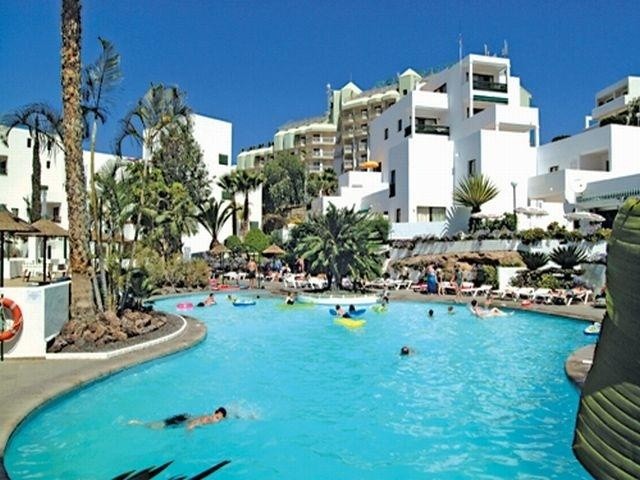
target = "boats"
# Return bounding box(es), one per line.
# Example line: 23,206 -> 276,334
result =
275,301 -> 315,311
334,317 -> 366,329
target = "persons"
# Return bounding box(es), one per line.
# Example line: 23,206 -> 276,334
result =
127,407 -> 226,434
197,255 -> 463,319
471,300 -> 514,319
400,346 -> 413,356
548,284 -> 606,306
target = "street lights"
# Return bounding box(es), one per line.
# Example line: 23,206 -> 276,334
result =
508,180 -> 517,214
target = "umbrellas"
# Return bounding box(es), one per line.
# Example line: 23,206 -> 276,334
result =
108,233 -> 132,263
90,229 -> 111,270
261,242 -> 288,264
0,204 -> 41,287
12,216 -> 70,283
209,243 -> 233,266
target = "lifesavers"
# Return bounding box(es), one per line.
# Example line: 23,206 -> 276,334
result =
0,297 -> 23,342
177,303 -> 193,308
334,315 -> 365,327
233,299 -> 256,306
